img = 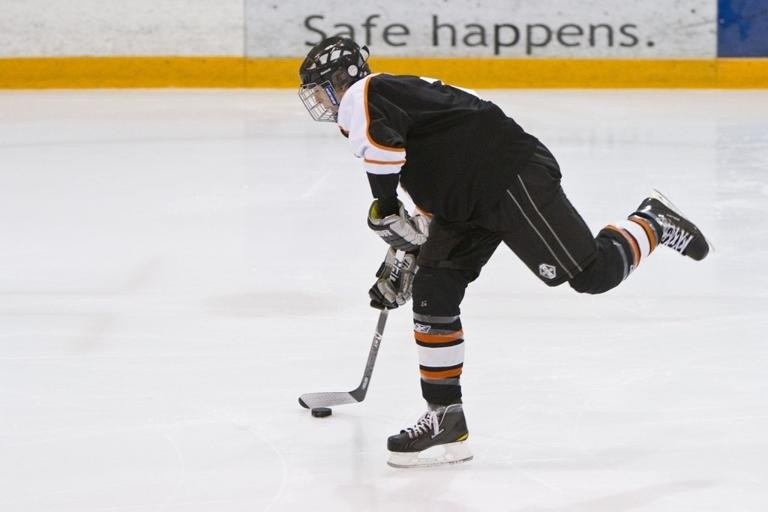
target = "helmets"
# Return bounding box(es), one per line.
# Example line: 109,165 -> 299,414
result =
300,37 -> 371,84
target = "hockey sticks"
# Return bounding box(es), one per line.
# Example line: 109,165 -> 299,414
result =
298,248 -> 405,409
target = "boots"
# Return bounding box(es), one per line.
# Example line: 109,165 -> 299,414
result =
628,197 -> 708,261
387,403 -> 468,452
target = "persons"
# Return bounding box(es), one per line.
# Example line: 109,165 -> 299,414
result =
299,37 -> 710,453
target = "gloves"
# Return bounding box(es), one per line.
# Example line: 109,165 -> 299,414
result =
367,200 -> 429,311
